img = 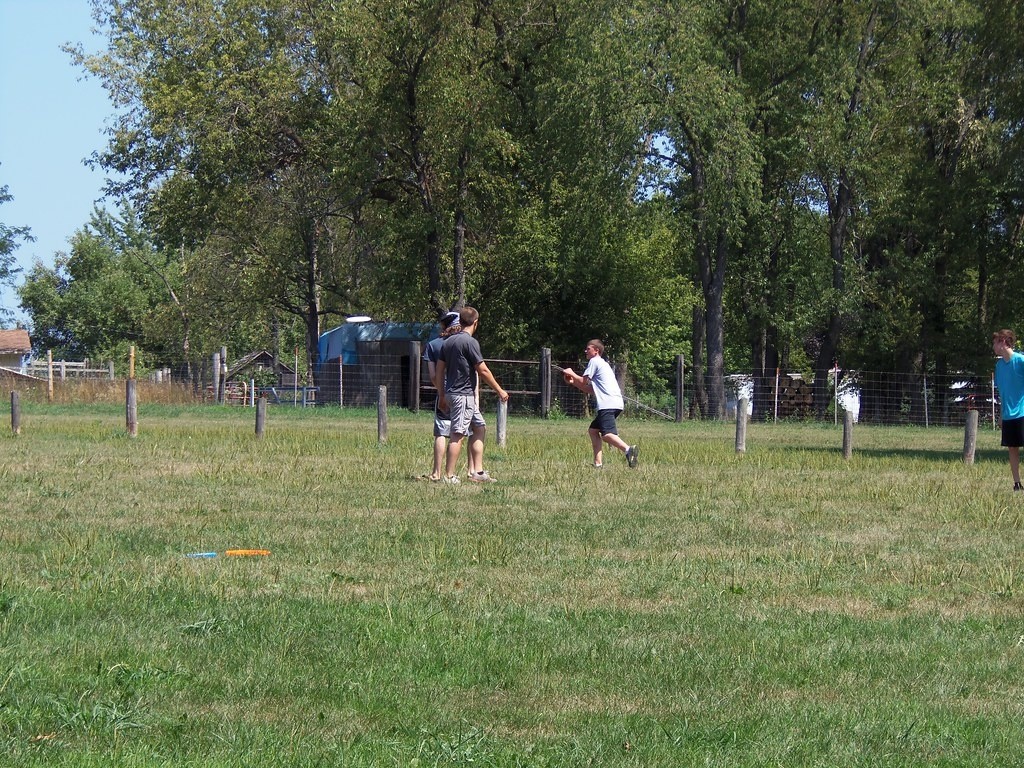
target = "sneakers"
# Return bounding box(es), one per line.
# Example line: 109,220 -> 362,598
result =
626,445 -> 638,468
422,475 -> 441,482
443,475 -> 461,484
467,473 -> 472,481
591,463 -> 603,470
472,473 -> 498,483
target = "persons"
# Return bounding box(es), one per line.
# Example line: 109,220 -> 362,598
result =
419,305 -> 512,486
562,338 -> 641,471
992,329 -> 1024,493
